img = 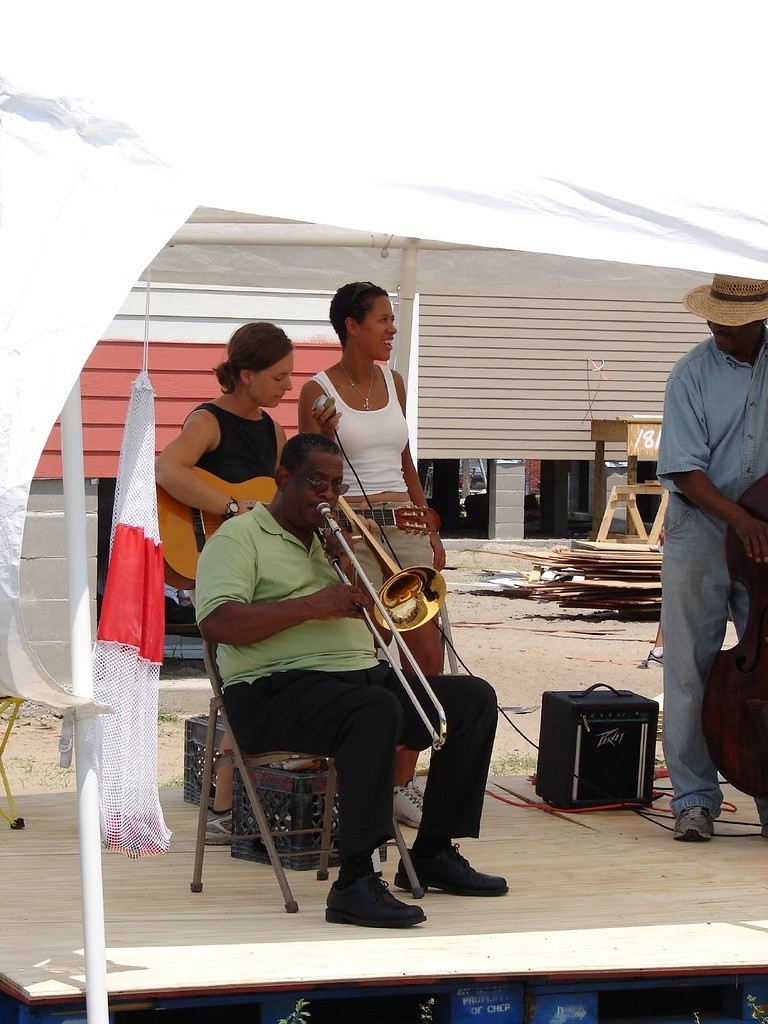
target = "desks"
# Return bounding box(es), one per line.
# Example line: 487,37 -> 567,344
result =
590,418 -> 662,541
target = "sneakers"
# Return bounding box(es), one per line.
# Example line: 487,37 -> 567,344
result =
392,769 -> 424,830
652,644 -> 664,658
673,805 -> 714,841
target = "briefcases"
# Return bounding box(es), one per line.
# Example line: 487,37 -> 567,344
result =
536,684 -> 661,807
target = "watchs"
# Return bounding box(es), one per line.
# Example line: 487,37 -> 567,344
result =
221,496 -> 241,520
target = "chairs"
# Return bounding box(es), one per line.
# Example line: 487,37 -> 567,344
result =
191,589 -> 425,913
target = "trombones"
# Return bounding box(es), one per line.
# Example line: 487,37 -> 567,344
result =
312,495 -> 449,749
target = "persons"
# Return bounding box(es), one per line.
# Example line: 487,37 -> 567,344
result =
297,280 -> 448,828
195,432 -> 509,928
155,323 -> 327,847
647,274 -> 768,840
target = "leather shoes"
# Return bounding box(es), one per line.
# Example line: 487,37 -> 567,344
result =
325,869 -> 428,928
393,841 -> 509,897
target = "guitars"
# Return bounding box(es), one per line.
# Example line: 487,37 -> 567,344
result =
156,465 -> 441,590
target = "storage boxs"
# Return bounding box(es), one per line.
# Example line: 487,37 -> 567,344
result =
184,715 -> 226,816
231,765 -> 387,872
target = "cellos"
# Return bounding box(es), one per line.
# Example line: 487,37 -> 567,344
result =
702,472 -> 768,801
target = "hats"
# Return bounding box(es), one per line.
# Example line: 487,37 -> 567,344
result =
682,272 -> 768,327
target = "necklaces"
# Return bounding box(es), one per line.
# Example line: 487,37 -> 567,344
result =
340,360 -> 374,411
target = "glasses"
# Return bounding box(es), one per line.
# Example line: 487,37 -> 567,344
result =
290,469 -> 350,495
